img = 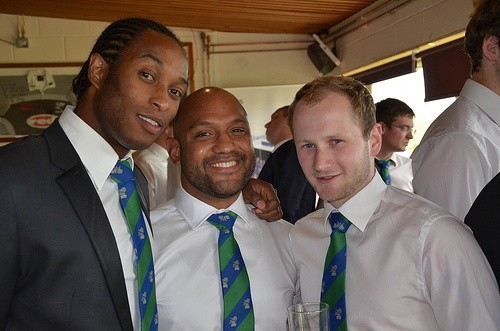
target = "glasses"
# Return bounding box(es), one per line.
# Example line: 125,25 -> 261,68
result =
385,124 -> 417,135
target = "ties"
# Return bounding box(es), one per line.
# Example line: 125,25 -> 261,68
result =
110,160 -> 158,331
205,210 -> 255,331
319,212 -> 352,331
374,158 -> 393,186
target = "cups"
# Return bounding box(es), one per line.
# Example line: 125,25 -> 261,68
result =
288,302 -> 330,331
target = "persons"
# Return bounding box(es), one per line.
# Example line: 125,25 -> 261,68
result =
136,121 -> 182,213
376,98 -> 420,192
288,77 -> 500,331
0,17 -> 189,331
146,86 -> 294,331
256,105 -> 315,224
412,1 -> 500,217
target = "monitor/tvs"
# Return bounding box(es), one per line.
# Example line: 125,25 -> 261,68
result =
307,33 -> 341,75
